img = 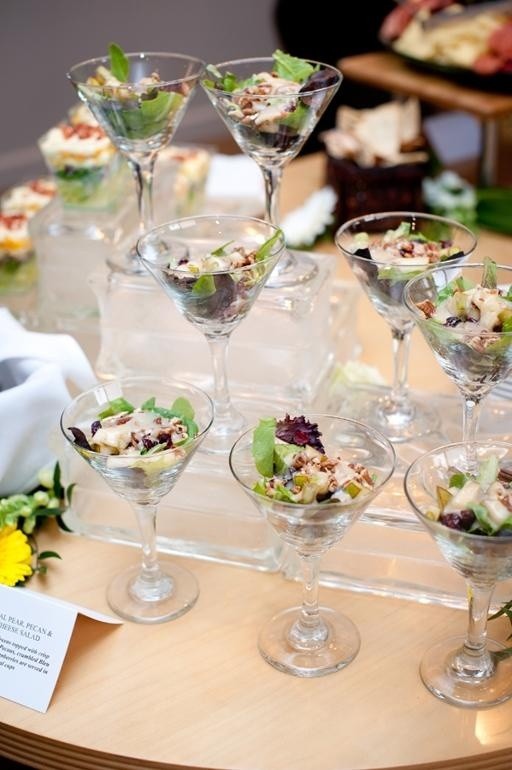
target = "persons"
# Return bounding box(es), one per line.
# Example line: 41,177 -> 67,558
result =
265,1 -> 448,164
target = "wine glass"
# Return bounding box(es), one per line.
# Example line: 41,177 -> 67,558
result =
203,55 -> 344,287
231,413 -> 397,675
136,214 -> 284,432
60,379 -> 216,625
405,262 -> 508,439
66,50 -> 201,271
405,440 -> 510,705
336,212 -> 481,435
